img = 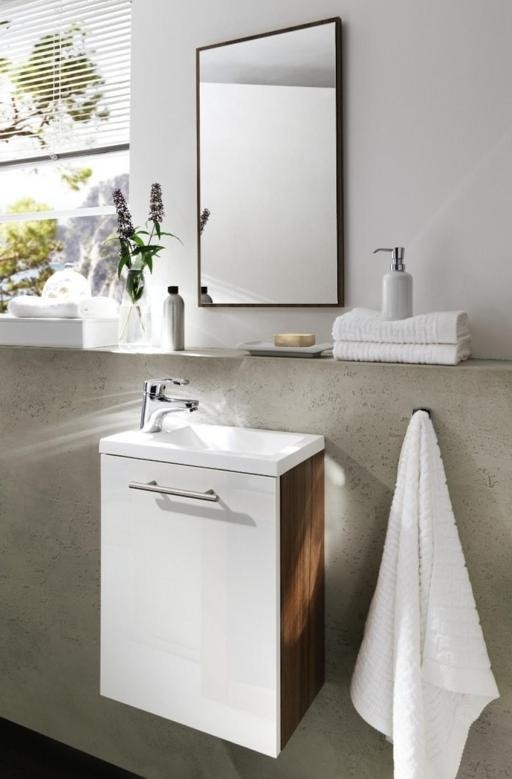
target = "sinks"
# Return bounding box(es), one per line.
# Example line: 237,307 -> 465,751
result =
98,423 -> 325,478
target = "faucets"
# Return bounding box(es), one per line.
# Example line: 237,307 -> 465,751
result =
141,377 -> 200,432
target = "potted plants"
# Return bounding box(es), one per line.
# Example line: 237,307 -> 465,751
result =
108,181 -> 181,350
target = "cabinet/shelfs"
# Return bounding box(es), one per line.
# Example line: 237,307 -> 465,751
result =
97,451 -> 324,759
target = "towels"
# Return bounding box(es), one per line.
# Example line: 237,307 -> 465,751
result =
12,295 -> 115,318
332,304 -> 470,364
349,414 -> 500,778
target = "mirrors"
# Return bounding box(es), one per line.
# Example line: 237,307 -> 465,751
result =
193,19 -> 344,307
